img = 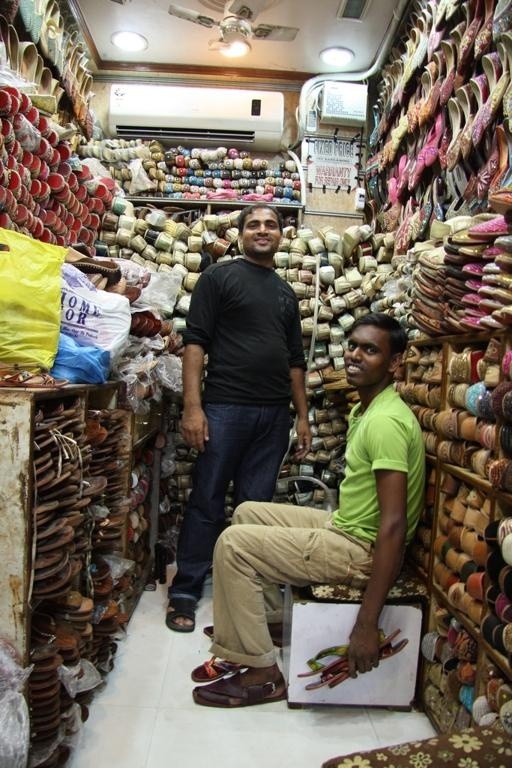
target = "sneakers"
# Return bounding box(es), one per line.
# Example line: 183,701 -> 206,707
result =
123,339 -> 185,406
276,340 -> 360,513
155,409 -> 196,583
1,0 -> 92,141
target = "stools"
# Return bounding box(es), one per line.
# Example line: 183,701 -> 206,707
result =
282,579 -> 422,711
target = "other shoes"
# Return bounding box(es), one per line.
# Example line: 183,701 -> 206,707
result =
65,248 -> 243,262
397,340 -> 512,731
271,224 -> 370,279
29,405 -> 151,763
371,3 -> 512,334
2,137 -> 301,202
287,280 -> 370,336
71,263 -> 200,336
1,203 -> 243,248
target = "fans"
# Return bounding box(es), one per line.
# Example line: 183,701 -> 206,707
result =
168,0 -> 299,42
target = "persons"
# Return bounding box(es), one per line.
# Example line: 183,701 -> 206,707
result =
193,313 -> 426,707
166,203 -> 313,632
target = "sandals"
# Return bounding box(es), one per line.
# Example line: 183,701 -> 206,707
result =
166,596 -> 291,706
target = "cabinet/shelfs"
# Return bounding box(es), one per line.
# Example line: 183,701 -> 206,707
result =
398,329 -> 512,739
0,381 -> 162,768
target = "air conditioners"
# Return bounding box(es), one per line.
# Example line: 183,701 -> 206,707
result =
110,82 -> 284,153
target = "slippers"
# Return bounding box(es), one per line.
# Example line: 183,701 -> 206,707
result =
298,629 -> 408,690
1,370 -> 69,388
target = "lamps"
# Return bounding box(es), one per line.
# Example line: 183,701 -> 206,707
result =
218,24 -> 251,56
